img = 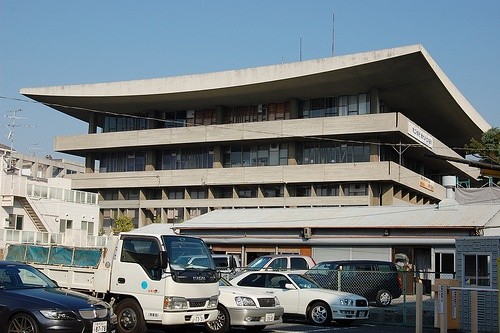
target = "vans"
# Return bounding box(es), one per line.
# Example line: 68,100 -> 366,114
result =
173,253 -> 243,276
303,260 -> 402,306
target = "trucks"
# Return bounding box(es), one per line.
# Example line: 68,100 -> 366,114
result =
11,233 -> 219,333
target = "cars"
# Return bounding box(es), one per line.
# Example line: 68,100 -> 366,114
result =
227,271 -> 369,326
0,259 -> 112,332
203,272 -> 284,331
242,255 -> 318,276
